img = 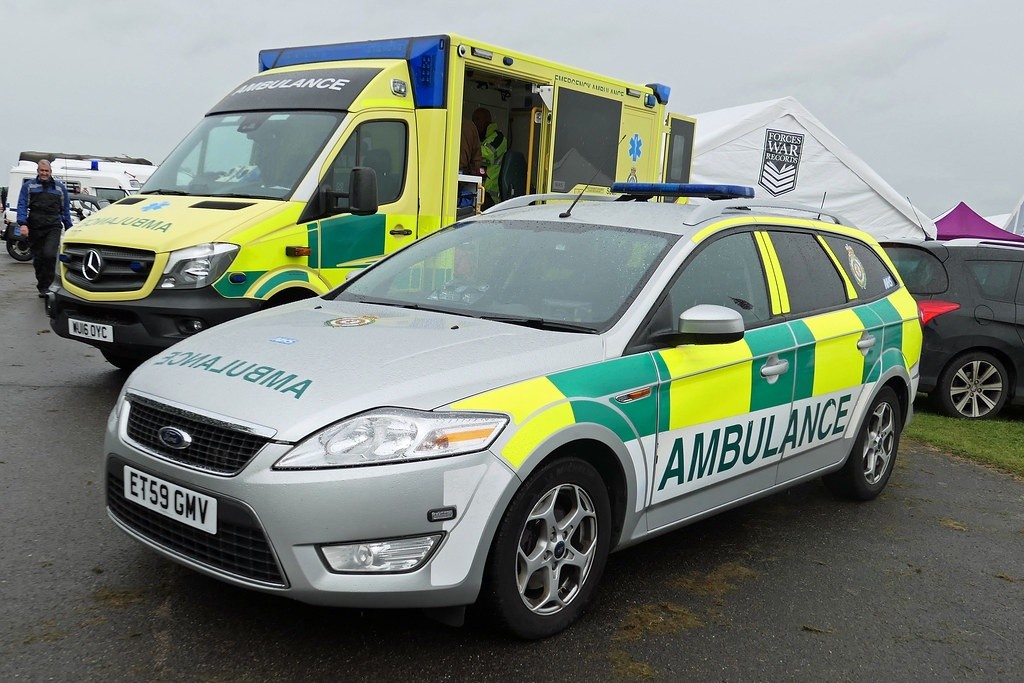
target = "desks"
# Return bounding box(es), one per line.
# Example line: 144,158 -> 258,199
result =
459,174 -> 485,212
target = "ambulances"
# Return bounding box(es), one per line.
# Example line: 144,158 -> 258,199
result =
46,32 -> 700,372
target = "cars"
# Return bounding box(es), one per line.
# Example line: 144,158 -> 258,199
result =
103,171 -> 924,646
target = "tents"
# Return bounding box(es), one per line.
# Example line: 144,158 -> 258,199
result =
667,98 -> 1024,245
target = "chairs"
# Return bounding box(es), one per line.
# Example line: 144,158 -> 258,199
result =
678,246 -> 751,328
364,149 -> 399,200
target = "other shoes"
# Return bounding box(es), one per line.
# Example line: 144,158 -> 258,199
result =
39,290 -> 50,298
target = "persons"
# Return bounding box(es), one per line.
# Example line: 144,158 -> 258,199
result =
458,105 -> 507,212
1,187 -> 7,213
17,159 -> 74,297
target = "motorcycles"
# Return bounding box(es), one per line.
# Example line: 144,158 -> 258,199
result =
0,201 -> 95,262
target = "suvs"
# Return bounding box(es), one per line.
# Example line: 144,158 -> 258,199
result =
862,235 -> 1024,422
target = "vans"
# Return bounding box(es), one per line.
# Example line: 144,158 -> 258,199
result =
5,150 -> 193,225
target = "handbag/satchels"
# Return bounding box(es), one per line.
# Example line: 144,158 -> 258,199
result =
4,222 -> 26,242
